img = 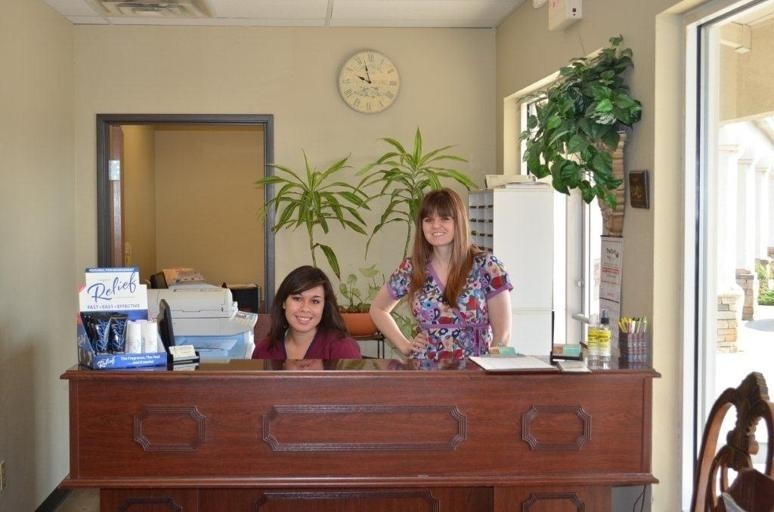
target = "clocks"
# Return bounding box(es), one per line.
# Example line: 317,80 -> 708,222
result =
337,49 -> 402,114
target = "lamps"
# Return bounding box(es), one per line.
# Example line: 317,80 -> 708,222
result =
532,1 -> 583,34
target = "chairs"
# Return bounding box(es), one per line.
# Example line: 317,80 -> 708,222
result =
692,372 -> 774,512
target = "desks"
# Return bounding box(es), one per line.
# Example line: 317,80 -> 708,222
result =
354,336 -> 385,359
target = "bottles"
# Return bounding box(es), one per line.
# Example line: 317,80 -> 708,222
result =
587,309 -> 612,371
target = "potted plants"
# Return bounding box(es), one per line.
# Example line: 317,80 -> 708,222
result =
516,33 -> 642,232
338,264 -> 386,337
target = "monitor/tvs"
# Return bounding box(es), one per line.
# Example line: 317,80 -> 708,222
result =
150,271 -> 168,289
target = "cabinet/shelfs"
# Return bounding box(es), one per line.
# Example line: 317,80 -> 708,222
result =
467,186 -> 566,356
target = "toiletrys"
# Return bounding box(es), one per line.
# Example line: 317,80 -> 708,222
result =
80,312 -> 128,353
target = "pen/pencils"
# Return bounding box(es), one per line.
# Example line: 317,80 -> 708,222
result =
618,314 -> 647,333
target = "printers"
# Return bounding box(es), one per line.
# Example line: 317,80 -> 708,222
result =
143,285 -> 258,360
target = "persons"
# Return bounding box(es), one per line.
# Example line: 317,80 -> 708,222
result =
267,358 -> 340,374
367,187 -> 517,361
249,264 -> 364,361
